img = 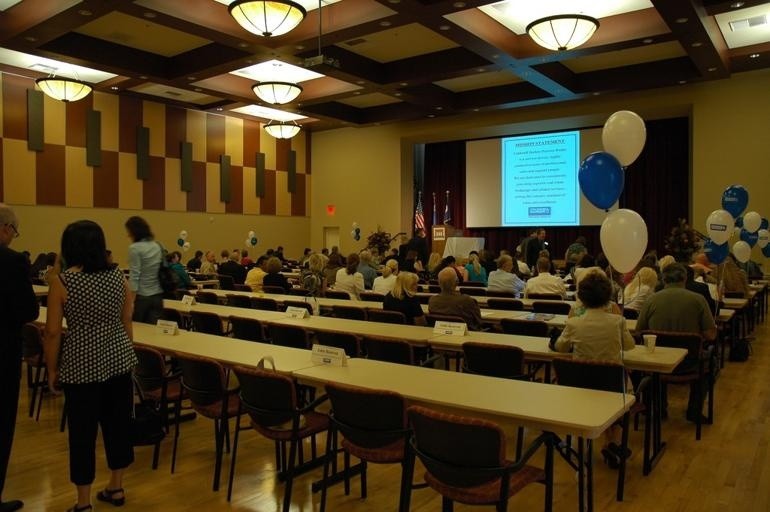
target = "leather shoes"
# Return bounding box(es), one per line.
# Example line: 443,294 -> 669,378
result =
0,500 -> 23,512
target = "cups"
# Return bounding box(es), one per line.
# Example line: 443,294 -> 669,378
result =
196,268 -> 199,273
643,334 -> 657,354
197,284 -> 203,292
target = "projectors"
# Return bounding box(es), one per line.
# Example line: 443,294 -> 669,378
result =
304,55 -> 340,69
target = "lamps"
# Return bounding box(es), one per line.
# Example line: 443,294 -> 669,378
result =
253,61 -> 303,108
522,13 -> 601,55
228,1 -> 308,41
262,109 -> 304,142
34,62 -> 97,105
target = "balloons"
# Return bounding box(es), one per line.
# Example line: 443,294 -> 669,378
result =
703,185 -> 770,264
601,109 -> 647,167
599,208 -> 649,275
578,150 -> 625,210
351,222 -> 360,241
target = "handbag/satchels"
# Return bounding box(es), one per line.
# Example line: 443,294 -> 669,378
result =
156,241 -> 179,295
549,327 -> 573,353
129,374 -> 167,447
728,337 -> 753,362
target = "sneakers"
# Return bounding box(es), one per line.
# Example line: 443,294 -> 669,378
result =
599,448 -> 619,470
608,441 -> 633,462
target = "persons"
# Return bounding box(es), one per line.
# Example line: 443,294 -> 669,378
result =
553,272 -> 636,469
46,219 -> 138,512
635,263 -> 718,425
125,216 -> 167,325
0,202 -> 40,512
23,250 -> 57,285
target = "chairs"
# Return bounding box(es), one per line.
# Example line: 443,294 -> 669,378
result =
19,235 -> 767,510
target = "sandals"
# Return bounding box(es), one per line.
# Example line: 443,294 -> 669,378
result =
97,487 -> 125,507
65,504 -> 93,512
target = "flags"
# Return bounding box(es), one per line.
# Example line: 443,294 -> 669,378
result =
433,197 -> 437,225
415,198 -> 430,239
444,197 -> 451,225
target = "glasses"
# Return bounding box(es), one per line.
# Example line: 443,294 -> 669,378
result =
5,223 -> 20,238
